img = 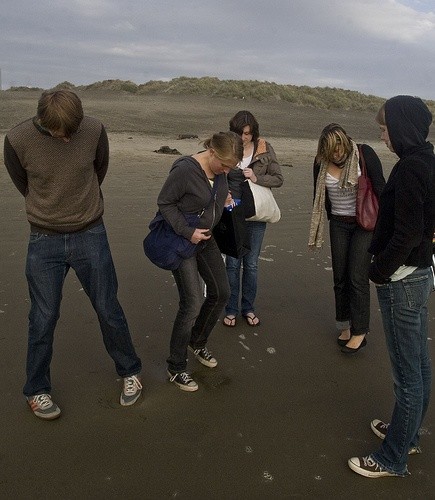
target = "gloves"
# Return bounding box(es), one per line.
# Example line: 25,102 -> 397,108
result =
368,261 -> 392,284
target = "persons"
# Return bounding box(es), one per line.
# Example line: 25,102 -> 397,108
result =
313,123 -> 386,356
348,95 -> 435,478
222,110 -> 283,329
142,131 -> 244,393
4,89 -> 142,420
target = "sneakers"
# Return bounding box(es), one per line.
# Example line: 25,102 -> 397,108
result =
187,345 -> 217,368
348,455 -> 408,478
371,419 -> 417,454
26,394 -> 61,419
166,367 -> 198,391
120,374 -> 142,406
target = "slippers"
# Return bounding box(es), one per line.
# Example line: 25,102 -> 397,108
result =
223,314 -> 236,327
245,312 -> 260,327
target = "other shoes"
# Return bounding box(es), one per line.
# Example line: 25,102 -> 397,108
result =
340,337 -> 367,353
337,331 -> 351,346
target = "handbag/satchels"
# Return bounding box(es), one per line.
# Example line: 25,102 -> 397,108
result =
355,144 -> 379,229
212,204 -> 251,259
142,213 -> 198,271
239,177 -> 281,223
